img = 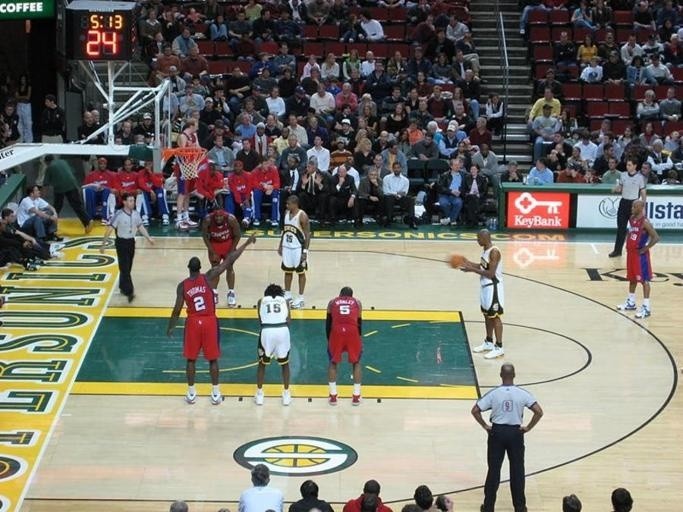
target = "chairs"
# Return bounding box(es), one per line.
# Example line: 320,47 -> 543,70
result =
521,1 -> 683,145
174,1 -> 463,89
167,160 -> 497,227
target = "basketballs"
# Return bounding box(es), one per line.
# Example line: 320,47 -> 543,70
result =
450,255 -> 465,270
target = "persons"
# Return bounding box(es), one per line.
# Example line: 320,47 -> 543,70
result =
394,129 -> 412,155
301,66 -> 325,94
610,488 -> 633,512
115,138 -> 122,145
572,132 -> 598,166
410,99 -> 433,129
0,103 -> 22,174
321,53 -> 340,79
373,131 -> 393,153
276,68 -> 297,97
358,93 -> 377,118
642,33 -> 664,64
140,9 -> 162,45
575,99 -> 590,131
328,165 -> 362,228
236,137 -> 260,171
385,103 -> 410,137
406,119 -> 423,145
646,139 -> 673,181
379,1 -> 405,10
569,129 -> 581,145
309,84 -> 335,114
81,157 -> 114,225
160,86 -> 179,121
631,1 -> 656,34
35,94 -> 67,187
625,55 -> 656,90
302,107 -> 327,129
244,0 -> 262,26
448,88 -> 468,119
279,154 -> 302,230
450,101 -> 472,135
329,1 -> 349,26
329,139 -> 354,172
266,145 -> 282,168
664,34 -> 682,69
360,494 -> 379,512
284,0 -> 307,23
387,51 -> 408,73
424,121 -> 443,144
152,45 -> 181,87
148,32 -> 170,62
617,200 -> 660,319
599,33 -> 619,60
249,52 -> 272,79
429,1 -> 450,20
243,85 -> 269,119
617,127 -> 636,145
471,362 -> 543,512
526,157 -> 554,186
425,28 -> 456,63
1,75 -> 12,102
187,8 -> 203,23
381,162 -> 419,230
14,73 -> 33,143
405,89 -> 422,113
459,229 -> 506,359
195,162 -> 226,222
306,0 -> 330,27
621,35 -> 642,65
671,136 -> 683,181
99,193 -> 155,303
182,109 -> 208,148
536,68 -> 564,104
566,146 -> 587,176
306,117 -> 329,147
92,110 -> 100,127
456,69 -> 482,123
436,496 -> 450,511
659,87 -> 682,121
339,14 -> 365,44
401,504 -> 421,512
181,46 -> 208,75
229,1 -> 246,20
273,8 -> 303,42
357,1 -> 378,8
461,163 -> 487,227
456,155 -> 466,173
109,159 -> 144,226
411,16 -> 436,42
40,154 -> 94,234
562,494 -> 582,512
519,0 -> 547,34
190,75 -> 205,95
159,66 -> 186,96
545,131 -> 573,168
447,120 -> 465,142
335,83 -> 358,110
147,0 -> 164,19
357,116 -> 369,131
333,119 -> 355,149
469,117 -> 492,152
272,128 -> 301,156
658,18 -> 675,43
0,124 -> 11,149
201,207 -> 242,307
592,143 -> 618,178
436,159 -> 466,225
143,132 -> 156,148
639,122 -> 660,150
300,55 -> 322,81
326,287 -> 363,406
262,114 -> 281,138
167,11 -> 184,40
251,122 -> 270,154
306,136 -> 330,171
119,122 -> 135,146
677,24 -> 683,42
240,99 -> 264,125
183,110 -> 210,149
174,118 -> 202,231
646,53 -> 674,83
452,50 -> 474,79
224,160 -> 253,229
285,86 -> 310,120
255,284 -> 291,407
342,49 -> 362,81
298,159 -> 329,228
470,143 -> 500,201
663,171 -> 680,185
179,83 -> 206,124
234,113 -> 256,138
601,156 -> 622,184
557,164 -> 587,183
549,0 -> 567,12
203,1 -> 223,24
263,0 -> 283,16
438,124 -> 461,160
170,503 -> 188,512
228,10 -> 253,61
532,104 -> 559,165
362,154 -> 391,179
355,167 -> 384,226
134,113 -> 155,137
281,134 -> 308,174
450,140 -> 472,173
359,104 -> 377,129
364,61 -> 392,99
266,87 -> 286,117
432,53 -> 454,81
225,66 -> 253,115
206,136 -> 235,175
333,104 -> 359,130
454,31 -> 475,54
528,86 -> 562,123
393,68 -> 415,96
427,85 -> 449,122
554,144 -> 565,155
636,90 -> 659,120
414,72 -> 433,100
554,31 -> 576,72
381,140 -> 408,178
414,484 -> 453,511
208,14 -> 227,42
597,132 -> 621,160
358,9 -> 383,40
266,111 -> 283,130
406,47 -> 432,78
361,51 -> 378,76
579,56 -> 604,83
345,68 -> 366,97
326,77 -> 341,94
162,4 -> 180,22
558,109 -> 574,139
591,116 -> 615,144
207,124 -> 235,151
577,35 -> 598,66
608,157 -> 646,257
200,98 -> 222,125
213,86 -> 231,128
331,157 -> 360,188
485,92 -> 504,136
664,130 -> 679,149
592,0 -> 613,29
137,161 -> 171,226
210,120 -> 234,137
1,208 -> 51,260
406,131 -> 439,162
287,111 -> 308,147
288,480 -> 335,512
238,464 -> 284,511
499,160 -> 523,182
274,42 -> 296,76
353,129 -> 369,141
17,184 -> 58,242
278,195 -> 311,310
545,151 -> 567,171
602,51 -> 626,85
657,0 -> 679,25
446,15 -> 472,51
382,87 -> 406,114
250,156 -> 282,226
342,480 -> 392,511
0,219 -> 40,272
570,1 -> 597,33
406,0 -> 430,27
167,231 -> 256,406
171,27 -> 200,58
77,112 -> 103,145
352,139 -> 377,173
249,8 -> 276,45
639,162 -> 659,185
251,68 -> 278,96
128,135 -> 153,162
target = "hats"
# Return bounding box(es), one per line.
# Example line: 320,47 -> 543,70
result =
295,86 -> 305,93
567,160 -> 579,169
447,125 -> 456,132
205,97 -> 214,103
169,66 -> 176,71
341,118 -> 350,124
543,103 -> 554,109
359,93 -> 372,101
335,138 -> 345,144
648,32 -> 658,41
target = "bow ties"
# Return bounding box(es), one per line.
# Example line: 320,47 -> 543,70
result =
472,178 -> 476,180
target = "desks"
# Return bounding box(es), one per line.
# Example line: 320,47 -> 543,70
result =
498,183 -> 683,234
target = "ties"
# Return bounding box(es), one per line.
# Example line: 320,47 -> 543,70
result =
291,172 -> 295,190
258,136 -> 262,158
310,176 -> 314,195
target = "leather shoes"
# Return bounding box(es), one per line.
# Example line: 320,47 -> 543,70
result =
377,220 -> 387,224
411,222 -> 418,230
608,250 -> 621,257
329,221 -> 335,229
127,292 -> 134,302
319,220 -> 326,228
353,223 -> 361,229
120,290 -> 128,296
386,222 -> 393,229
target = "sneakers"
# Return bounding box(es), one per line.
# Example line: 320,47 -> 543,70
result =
42,241 -> 50,246
212,293 -> 219,303
440,218 -> 450,226
292,299 -> 304,308
271,220 -> 278,227
228,293 -> 236,307
451,221 -> 457,226
30,257 -> 46,264
51,243 -> 65,250
183,218 -> 199,228
48,235 -> 64,241
484,348 -> 505,357
282,395 -> 290,405
174,218 -> 188,230
328,395 -> 338,404
254,220 -> 262,227
143,216 -> 149,226
284,297 -> 292,306
473,342 -> 492,351
101,218 -> 111,226
186,394 -> 197,403
352,395 -> 361,407
162,215 -> 170,225
210,393 -> 221,404
27,261 -> 39,270
617,300 -> 635,310
84,220 -> 94,234
241,217 -> 250,229
50,251 -> 63,258
635,306 -> 650,317
254,396 -> 263,405
